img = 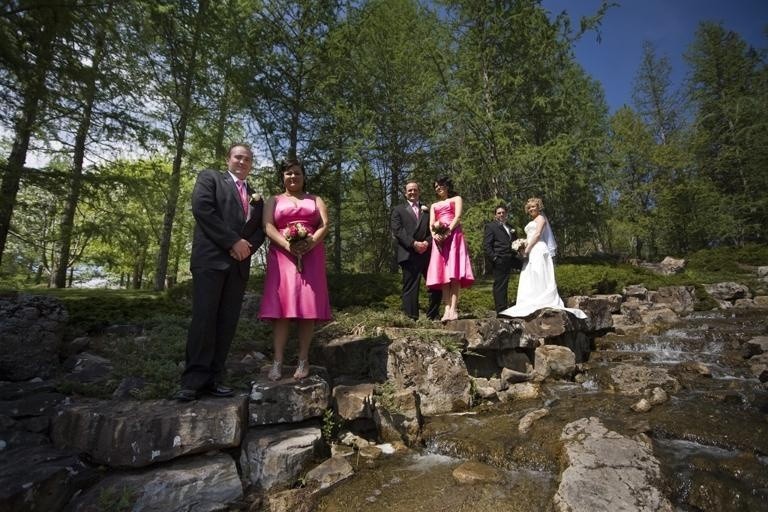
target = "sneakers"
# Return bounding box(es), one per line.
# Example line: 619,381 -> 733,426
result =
441,311 -> 458,323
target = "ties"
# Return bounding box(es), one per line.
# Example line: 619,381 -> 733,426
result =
235,179 -> 248,219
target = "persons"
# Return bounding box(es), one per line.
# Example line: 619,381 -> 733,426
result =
390,182 -> 443,322
497,198 -> 588,319
175,145 -> 266,401
263,159 -> 332,381
425,176 -> 475,322
483,205 -> 519,316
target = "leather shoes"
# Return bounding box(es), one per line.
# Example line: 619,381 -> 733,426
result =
172,391 -> 201,400
203,386 -> 234,397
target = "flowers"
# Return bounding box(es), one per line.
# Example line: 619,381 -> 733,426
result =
429,221 -> 453,254
513,238 -> 529,260
419,202 -> 431,213
283,219 -> 311,273
249,191 -> 261,206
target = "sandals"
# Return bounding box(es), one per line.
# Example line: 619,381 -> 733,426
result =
268,360 -> 284,381
293,359 -> 310,380
